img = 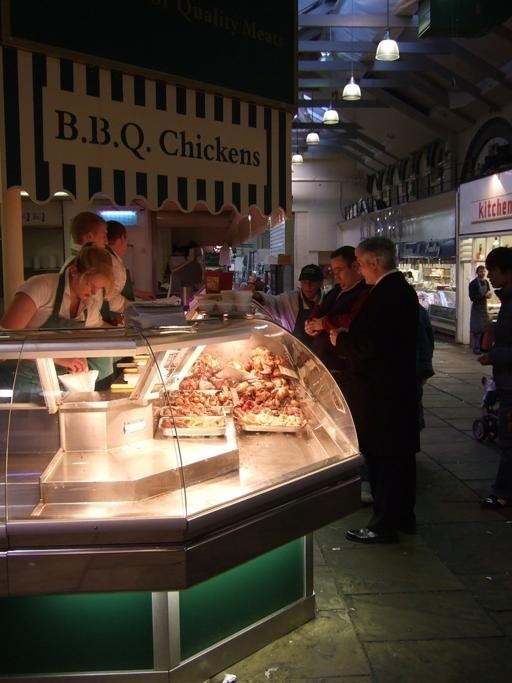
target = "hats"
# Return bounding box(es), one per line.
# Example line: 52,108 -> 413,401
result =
298,264 -> 323,282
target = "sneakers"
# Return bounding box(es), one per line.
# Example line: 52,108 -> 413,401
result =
480,495 -> 509,510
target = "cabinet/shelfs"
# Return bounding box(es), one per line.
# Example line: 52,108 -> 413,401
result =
423,264 -> 452,283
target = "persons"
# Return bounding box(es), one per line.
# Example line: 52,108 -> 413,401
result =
254,264 -> 327,346
330,237 -> 424,542
404,271 -> 413,282
304,246 -> 368,481
469,247 -> 512,510
0,210 -> 157,403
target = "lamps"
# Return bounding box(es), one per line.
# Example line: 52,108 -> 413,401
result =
375,0 -> 400,61
306,89 -> 320,144
292,116 -> 303,163
343,0 -> 365,100
322,44 -> 339,124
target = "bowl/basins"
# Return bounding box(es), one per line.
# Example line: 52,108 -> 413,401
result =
200,290 -> 254,311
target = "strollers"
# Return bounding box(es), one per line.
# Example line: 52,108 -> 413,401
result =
473,375 -> 502,447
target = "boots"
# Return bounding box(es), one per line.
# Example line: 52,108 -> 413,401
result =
474,335 -> 481,353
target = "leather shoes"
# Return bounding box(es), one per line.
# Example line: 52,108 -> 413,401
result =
344,511 -> 417,544
